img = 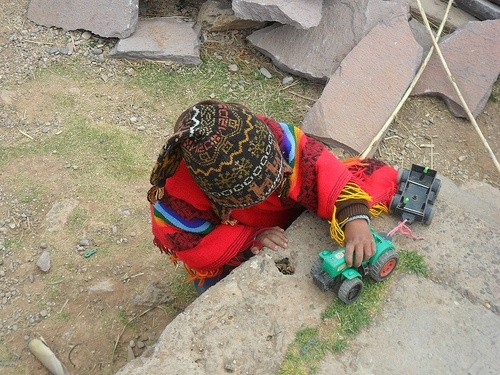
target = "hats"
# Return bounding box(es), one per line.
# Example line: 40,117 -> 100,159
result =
146,100 -> 294,224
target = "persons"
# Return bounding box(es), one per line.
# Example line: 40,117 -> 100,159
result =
148,99 -> 377,297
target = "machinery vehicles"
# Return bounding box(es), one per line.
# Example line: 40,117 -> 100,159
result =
310,228 -> 400,304
